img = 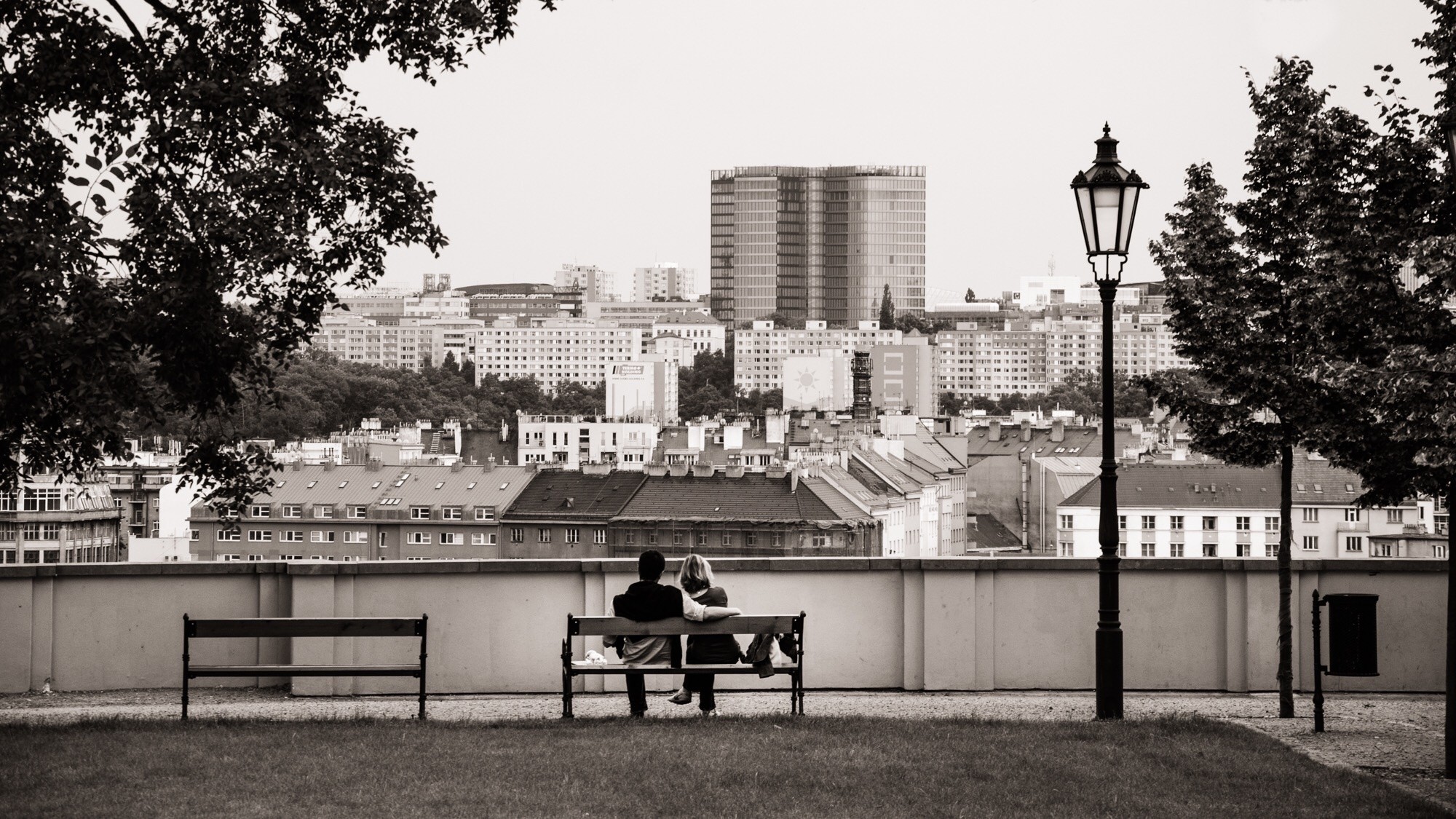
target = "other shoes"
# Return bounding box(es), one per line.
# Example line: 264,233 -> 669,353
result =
703,710 -> 716,717
632,711 -> 644,717
667,689 -> 691,704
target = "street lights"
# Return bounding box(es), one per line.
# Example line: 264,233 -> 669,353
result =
1069,118 -> 1152,727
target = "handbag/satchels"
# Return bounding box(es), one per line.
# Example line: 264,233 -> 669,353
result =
622,635 -> 671,666
778,634 -> 798,664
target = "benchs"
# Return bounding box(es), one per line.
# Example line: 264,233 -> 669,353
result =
560,611 -> 807,719
182,613 -> 429,726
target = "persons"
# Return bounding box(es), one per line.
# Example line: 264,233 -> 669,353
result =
664,553 -> 740,713
602,551 -> 743,718
